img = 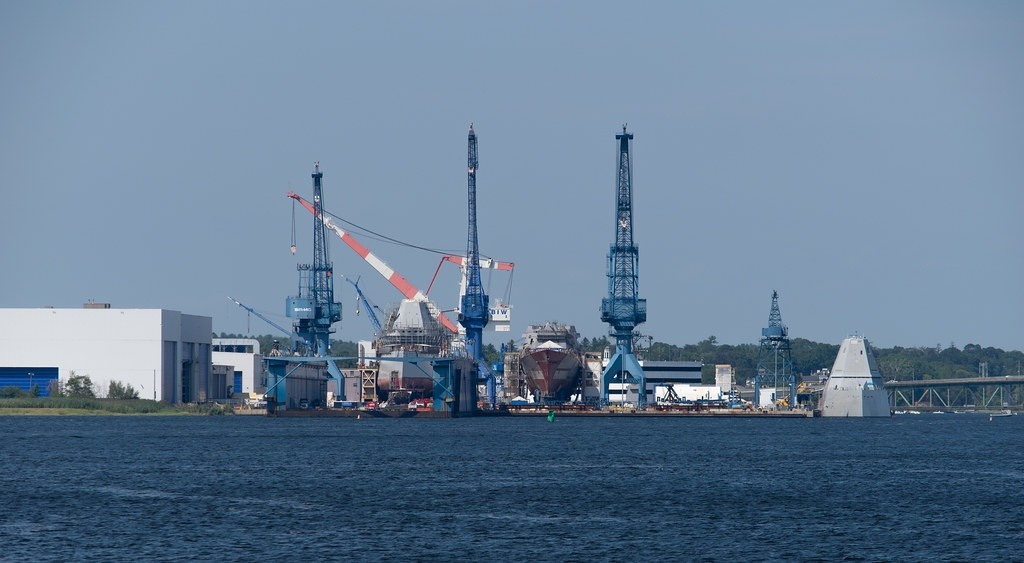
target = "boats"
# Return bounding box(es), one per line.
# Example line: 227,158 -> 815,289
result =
908,410 -> 921,414
819,330 -> 892,417
933,410 -> 945,414
377,299 -> 449,406
955,404 -> 978,414
518,320 -> 583,405
990,407 -> 1013,417
895,410 -> 907,414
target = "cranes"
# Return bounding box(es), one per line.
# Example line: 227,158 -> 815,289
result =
432,121 -> 498,410
264,161 -> 345,404
285,192 -> 516,336
753,292 -> 798,408
226,296 -> 292,336
339,274 -> 382,333
598,122 -> 651,411
